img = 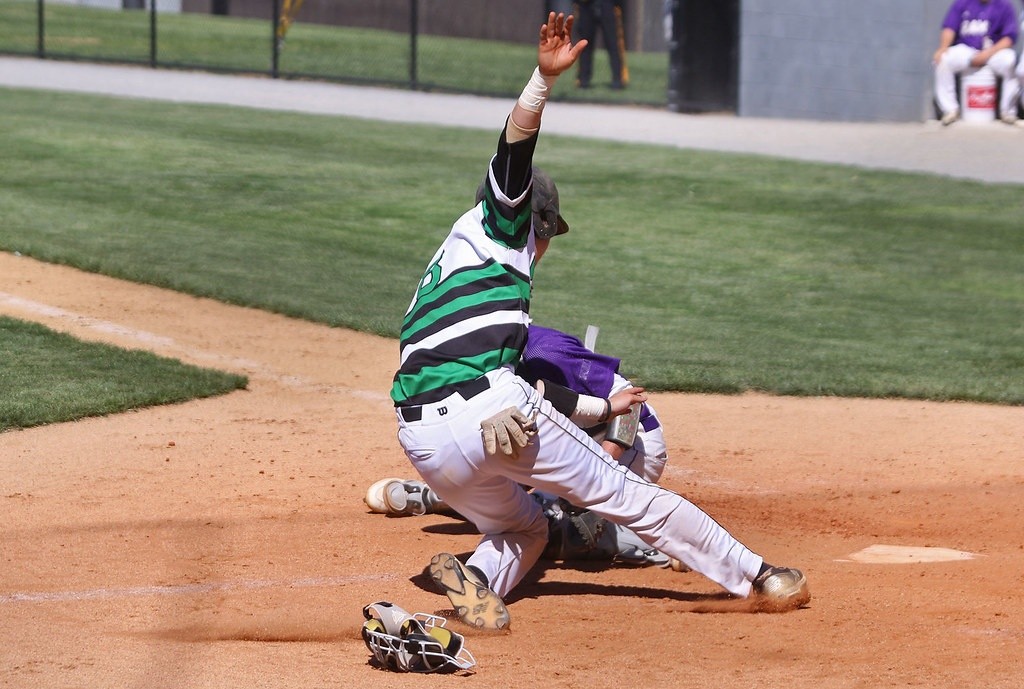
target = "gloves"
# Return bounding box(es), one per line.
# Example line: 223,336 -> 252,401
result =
481,407 -> 536,460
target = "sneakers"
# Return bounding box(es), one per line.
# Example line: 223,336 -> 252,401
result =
753,566 -> 805,607
363,477 -> 450,517
670,556 -> 689,572
430,552 -> 510,631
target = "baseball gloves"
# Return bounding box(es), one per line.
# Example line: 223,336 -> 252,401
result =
562,503 -> 619,573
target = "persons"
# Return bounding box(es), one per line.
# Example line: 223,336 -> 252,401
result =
570,0 -> 629,88
933,0 -> 1024,126
365,13 -> 808,632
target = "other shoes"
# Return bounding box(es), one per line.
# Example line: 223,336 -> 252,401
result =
941,107 -> 961,126
1003,113 -> 1024,129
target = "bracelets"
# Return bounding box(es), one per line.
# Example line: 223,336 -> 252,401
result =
598,397 -> 611,423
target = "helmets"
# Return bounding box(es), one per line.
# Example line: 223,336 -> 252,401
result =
475,162 -> 570,240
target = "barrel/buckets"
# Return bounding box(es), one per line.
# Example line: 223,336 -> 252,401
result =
960,65 -> 997,120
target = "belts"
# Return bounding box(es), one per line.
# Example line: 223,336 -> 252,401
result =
400,375 -> 491,422
